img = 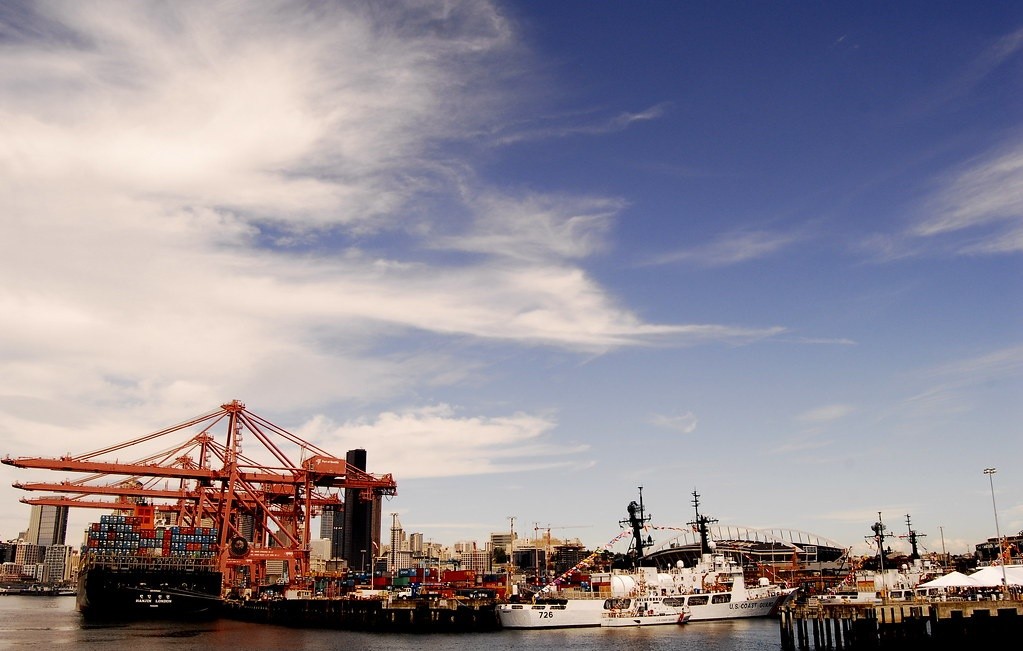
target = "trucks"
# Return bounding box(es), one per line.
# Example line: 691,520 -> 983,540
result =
397,584 -> 442,601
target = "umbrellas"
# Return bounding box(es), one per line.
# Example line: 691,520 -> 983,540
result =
917,567 -> 1023,594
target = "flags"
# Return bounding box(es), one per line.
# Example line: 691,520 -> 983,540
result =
533,530 -> 640,611
991,543 -> 1023,567
644,523 -> 877,598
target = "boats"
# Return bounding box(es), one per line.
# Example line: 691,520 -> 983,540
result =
811,512 -> 945,605
600,593 -> 693,626
494,485 -> 800,629
74,556 -> 223,623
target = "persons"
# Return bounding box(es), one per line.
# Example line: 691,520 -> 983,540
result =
678,608 -> 685,622
637,602 -> 645,617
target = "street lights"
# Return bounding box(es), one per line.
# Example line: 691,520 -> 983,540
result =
372,554 -> 375,590
983,467 -> 1010,600
361,550 -> 366,572
333,526 -> 342,570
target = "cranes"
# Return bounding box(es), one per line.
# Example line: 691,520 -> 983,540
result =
535,522 -> 595,546
1,397 -> 398,593
